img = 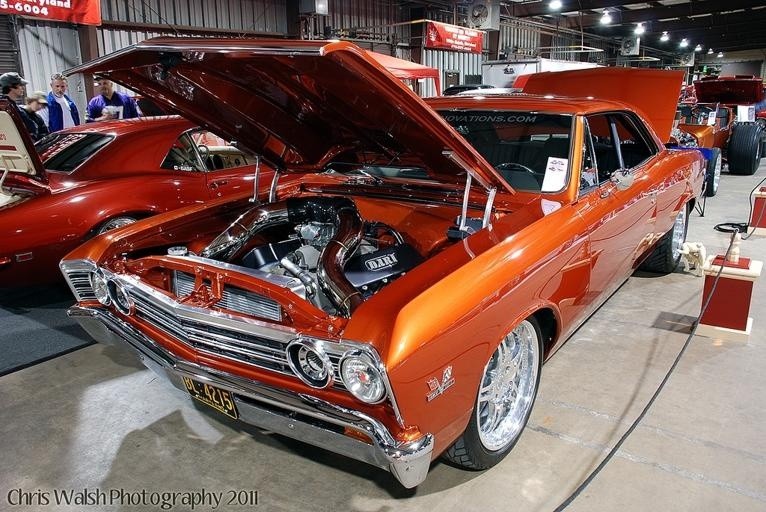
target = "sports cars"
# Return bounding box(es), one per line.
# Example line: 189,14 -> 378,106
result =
668,102 -> 762,197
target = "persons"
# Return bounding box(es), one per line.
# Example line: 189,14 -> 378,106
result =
20,91 -> 49,141
84,76 -> 138,123
1,72 -> 38,143
36,73 -> 79,134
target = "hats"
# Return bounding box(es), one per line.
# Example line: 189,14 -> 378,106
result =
28,91 -> 49,106
94,74 -> 103,81
0,71 -> 28,89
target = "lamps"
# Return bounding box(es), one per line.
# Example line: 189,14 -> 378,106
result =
694,44 -> 702,52
717,50 -> 723,58
659,31 -> 669,41
600,8 -> 612,25
679,38 -> 688,47
707,48 -> 714,54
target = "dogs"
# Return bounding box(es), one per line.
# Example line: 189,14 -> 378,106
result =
676,242 -> 706,277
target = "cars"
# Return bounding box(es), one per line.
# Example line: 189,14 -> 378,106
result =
0,95 -> 305,291
443,84 -> 495,97
56,35 -> 706,498
452,87 -> 524,97
679,74 -> 766,159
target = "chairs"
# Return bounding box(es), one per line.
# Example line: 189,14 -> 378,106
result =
468,122 -> 519,169
533,137 -> 570,177
